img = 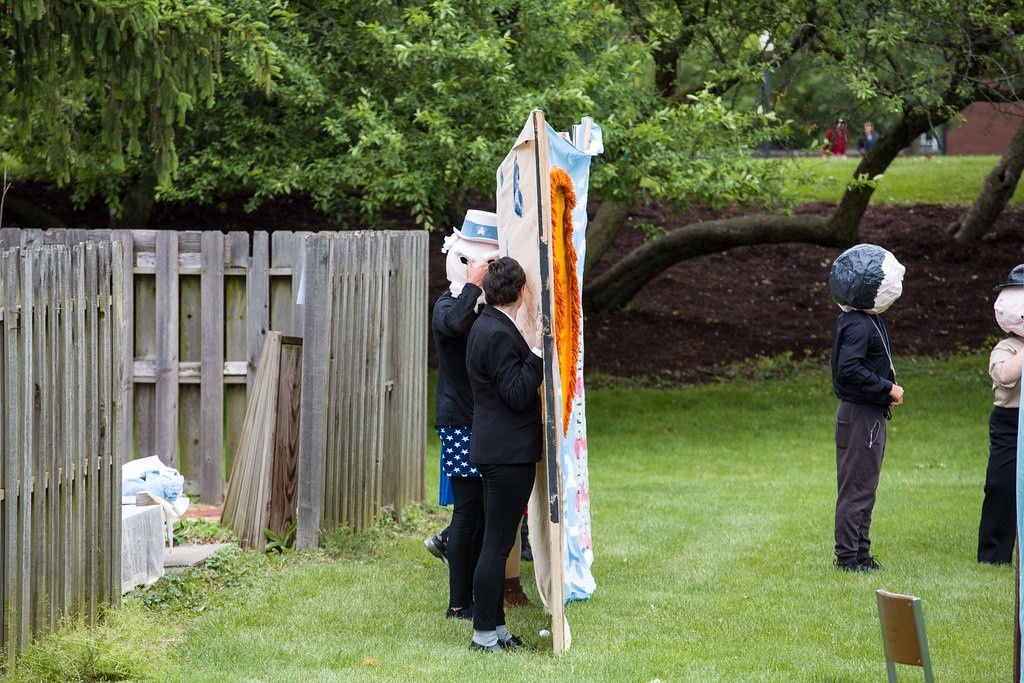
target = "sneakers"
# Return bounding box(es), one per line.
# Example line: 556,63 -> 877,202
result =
424,533 -> 449,566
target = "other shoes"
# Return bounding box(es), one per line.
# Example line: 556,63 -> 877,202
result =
445,601 -> 475,621
836,555 -> 885,573
469,634 -> 534,655
522,549 -> 533,561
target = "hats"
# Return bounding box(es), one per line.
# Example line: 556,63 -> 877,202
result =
452,210 -> 498,245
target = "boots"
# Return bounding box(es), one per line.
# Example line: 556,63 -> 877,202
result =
503,576 -> 536,609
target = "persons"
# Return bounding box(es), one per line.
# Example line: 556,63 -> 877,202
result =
520,515 -> 533,562
435,285 -> 500,621
425,521 -> 450,566
466,256 -> 544,650
823,118 -> 850,157
505,516 -> 537,609
828,311 -> 904,572
978,335 -> 1024,566
858,121 -> 879,157
